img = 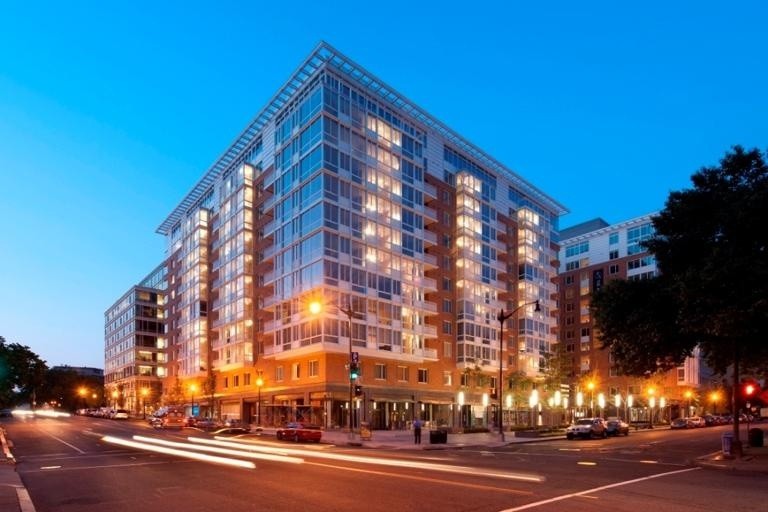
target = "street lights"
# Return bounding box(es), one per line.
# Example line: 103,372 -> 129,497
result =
140,387 -> 149,418
647,386 -> 656,430
711,393 -> 720,413
191,384 -> 196,414
112,390 -> 118,410
307,298 -> 354,439
683,389 -> 693,417
497,299 -> 542,443
586,381 -> 596,417
257,378 -> 262,425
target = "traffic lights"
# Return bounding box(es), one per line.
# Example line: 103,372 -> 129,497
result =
349,364 -> 358,380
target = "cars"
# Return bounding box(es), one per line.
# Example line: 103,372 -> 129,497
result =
671,414 -> 754,428
148,407 -> 184,429
566,416 -> 630,439
185,416 -> 251,430
78,407 -> 130,420
276,421 -> 323,444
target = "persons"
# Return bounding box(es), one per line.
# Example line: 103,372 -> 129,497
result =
413,417 -> 421,444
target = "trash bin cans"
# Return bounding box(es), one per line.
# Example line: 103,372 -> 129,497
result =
722,432 -> 737,455
429,429 -> 447,444
748,427 -> 763,447
